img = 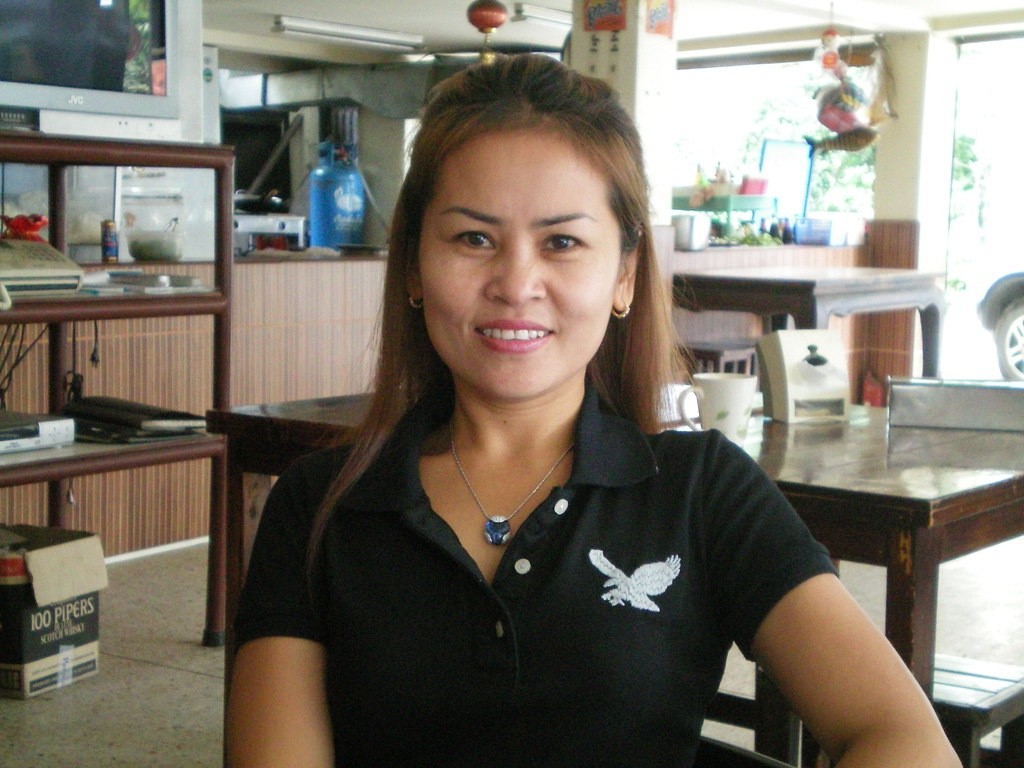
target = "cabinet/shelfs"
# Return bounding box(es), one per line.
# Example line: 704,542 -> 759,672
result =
0,129 -> 238,646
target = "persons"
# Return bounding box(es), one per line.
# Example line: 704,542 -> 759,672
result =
226,54 -> 964,768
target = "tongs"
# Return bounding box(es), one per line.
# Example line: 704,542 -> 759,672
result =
160,217 -> 179,233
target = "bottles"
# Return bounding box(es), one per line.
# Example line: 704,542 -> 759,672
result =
100,219 -> 119,264
861,370 -> 884,406
758,215 -> 831,246
695,164 -> 710,188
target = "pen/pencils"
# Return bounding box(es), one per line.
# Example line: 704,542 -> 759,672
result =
82,287 -> 128,293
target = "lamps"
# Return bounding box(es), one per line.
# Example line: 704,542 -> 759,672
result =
269,13 -> 424,54
509,3 -> 573,30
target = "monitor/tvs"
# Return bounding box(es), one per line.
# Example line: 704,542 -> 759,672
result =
0,0 -> 206,146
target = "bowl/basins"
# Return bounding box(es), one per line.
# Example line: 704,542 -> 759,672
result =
122,229 -> 186,261
67,242 -> 102,265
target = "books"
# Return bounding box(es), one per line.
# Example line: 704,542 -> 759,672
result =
58,396 -> 207,454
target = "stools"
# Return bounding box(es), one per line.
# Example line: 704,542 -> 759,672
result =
687,338 -> 760,378
930,652 -> 1024,768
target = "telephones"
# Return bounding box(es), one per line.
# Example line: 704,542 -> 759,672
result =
0,238 -> 84,295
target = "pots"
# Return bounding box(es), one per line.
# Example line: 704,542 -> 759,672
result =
235,189 -> 283,214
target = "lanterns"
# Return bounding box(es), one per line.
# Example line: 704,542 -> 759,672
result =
466,0 -> 508,45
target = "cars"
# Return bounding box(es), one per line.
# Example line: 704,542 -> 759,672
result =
942,264 -> 1024,386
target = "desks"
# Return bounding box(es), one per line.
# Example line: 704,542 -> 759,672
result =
205,393 -> 1024,767
670,266 -> 948,381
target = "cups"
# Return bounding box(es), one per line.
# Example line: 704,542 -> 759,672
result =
677,372 -> 757,445
672,210 -> 712,251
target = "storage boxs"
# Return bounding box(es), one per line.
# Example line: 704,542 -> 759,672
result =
1,522 -> 108,700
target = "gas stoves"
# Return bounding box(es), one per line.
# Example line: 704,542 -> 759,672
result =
234,212 -> 306,249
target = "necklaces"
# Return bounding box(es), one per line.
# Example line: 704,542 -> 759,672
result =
448,435 -> 576,545
